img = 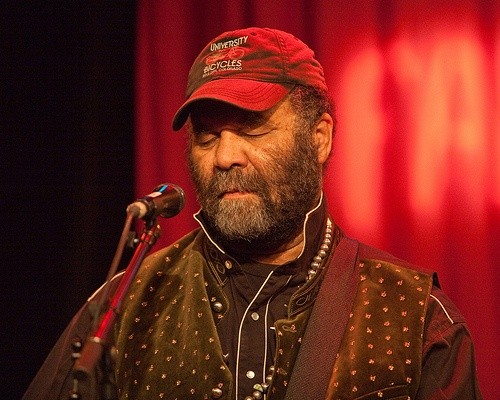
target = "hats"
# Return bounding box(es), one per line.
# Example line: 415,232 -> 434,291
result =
172,26 -> 329,129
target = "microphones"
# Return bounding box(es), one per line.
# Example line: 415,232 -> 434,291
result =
127,182 -> 186,218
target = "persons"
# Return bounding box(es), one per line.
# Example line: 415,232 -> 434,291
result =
21,27 -> 486,400
